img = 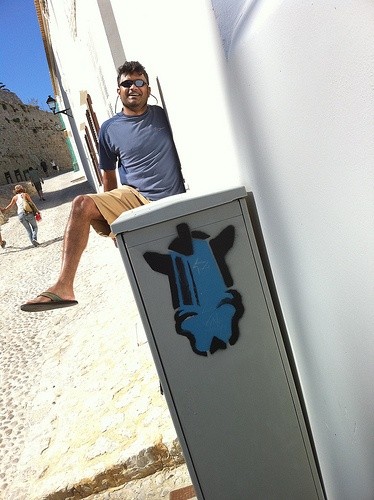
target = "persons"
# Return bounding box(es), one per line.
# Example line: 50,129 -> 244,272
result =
40,159 -> 50,177
28,167 -> 46,201
21,61 -> 186,312
0,184 -> 42,248
50,159 -> 58,173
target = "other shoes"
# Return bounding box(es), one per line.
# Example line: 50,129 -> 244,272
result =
1,241 -> 6,248
42,197 -> 45,201
32,237 -> 39,247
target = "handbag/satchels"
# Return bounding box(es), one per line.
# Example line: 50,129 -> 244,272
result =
23,201 -> 33,214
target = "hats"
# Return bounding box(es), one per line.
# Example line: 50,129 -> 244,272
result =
13,184 -> 27,194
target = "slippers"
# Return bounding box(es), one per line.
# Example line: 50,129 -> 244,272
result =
21,291 -> 78,313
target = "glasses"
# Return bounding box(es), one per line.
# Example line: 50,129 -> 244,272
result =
119,78 -> 149,88
